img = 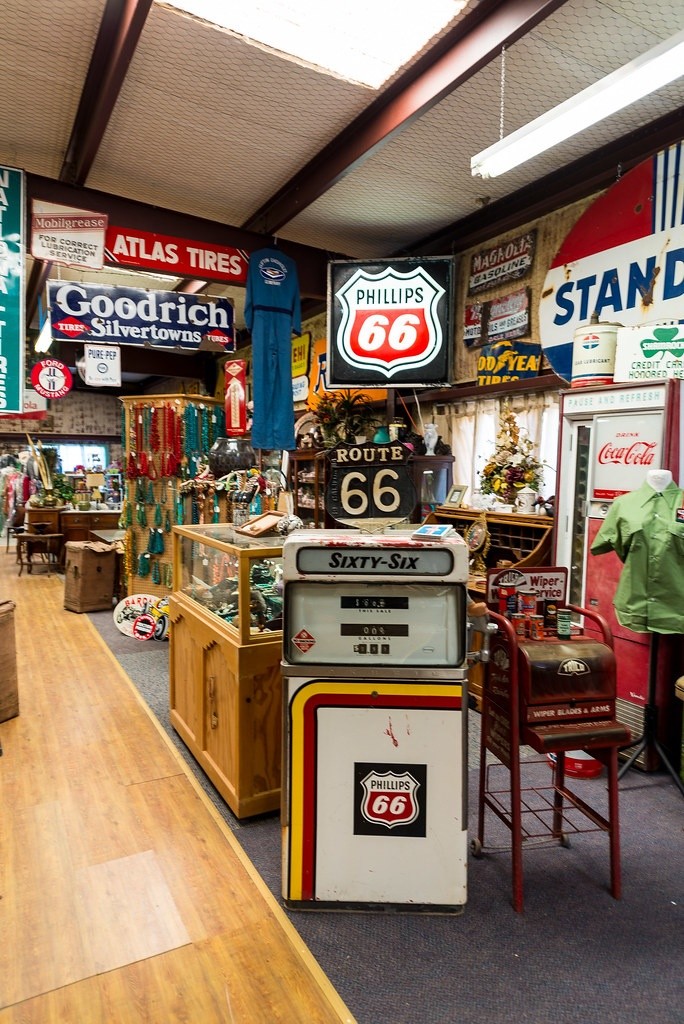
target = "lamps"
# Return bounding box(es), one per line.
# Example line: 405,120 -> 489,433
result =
36,295 -> 54,353
471,28 -> 684,179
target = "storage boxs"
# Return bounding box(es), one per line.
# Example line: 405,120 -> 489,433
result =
0,596 -> 19,723
63,541 -> 115,613
613,327 -> 684,383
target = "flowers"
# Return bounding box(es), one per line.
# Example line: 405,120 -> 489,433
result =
477,402 -> 545,503
304,391 -> 340,426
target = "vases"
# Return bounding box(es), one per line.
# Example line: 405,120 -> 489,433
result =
424,424 -> 440,457
356,436 -> 367,444
389,424 -> 407,441
208,438 -> 253,478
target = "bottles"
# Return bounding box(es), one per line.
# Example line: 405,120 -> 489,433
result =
389,417 -> 407,442
423,424 -> 438,456
374,426 -> 389,444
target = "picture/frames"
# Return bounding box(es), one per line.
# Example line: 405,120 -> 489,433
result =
235,511 -> 287,538
445,483 -> 468,509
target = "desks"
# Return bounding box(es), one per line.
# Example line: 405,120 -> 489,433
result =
13,534 -> 64,577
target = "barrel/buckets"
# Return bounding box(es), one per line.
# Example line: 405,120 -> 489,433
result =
570,323 -> 626,389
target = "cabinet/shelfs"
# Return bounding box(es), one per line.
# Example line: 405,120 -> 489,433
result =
288,451 -> 455,529
61,510 -> 123,541
25,502 -> 61,571
434,506 -> 555,714
89,529 -> 127,597
170,522 -> 287,818
